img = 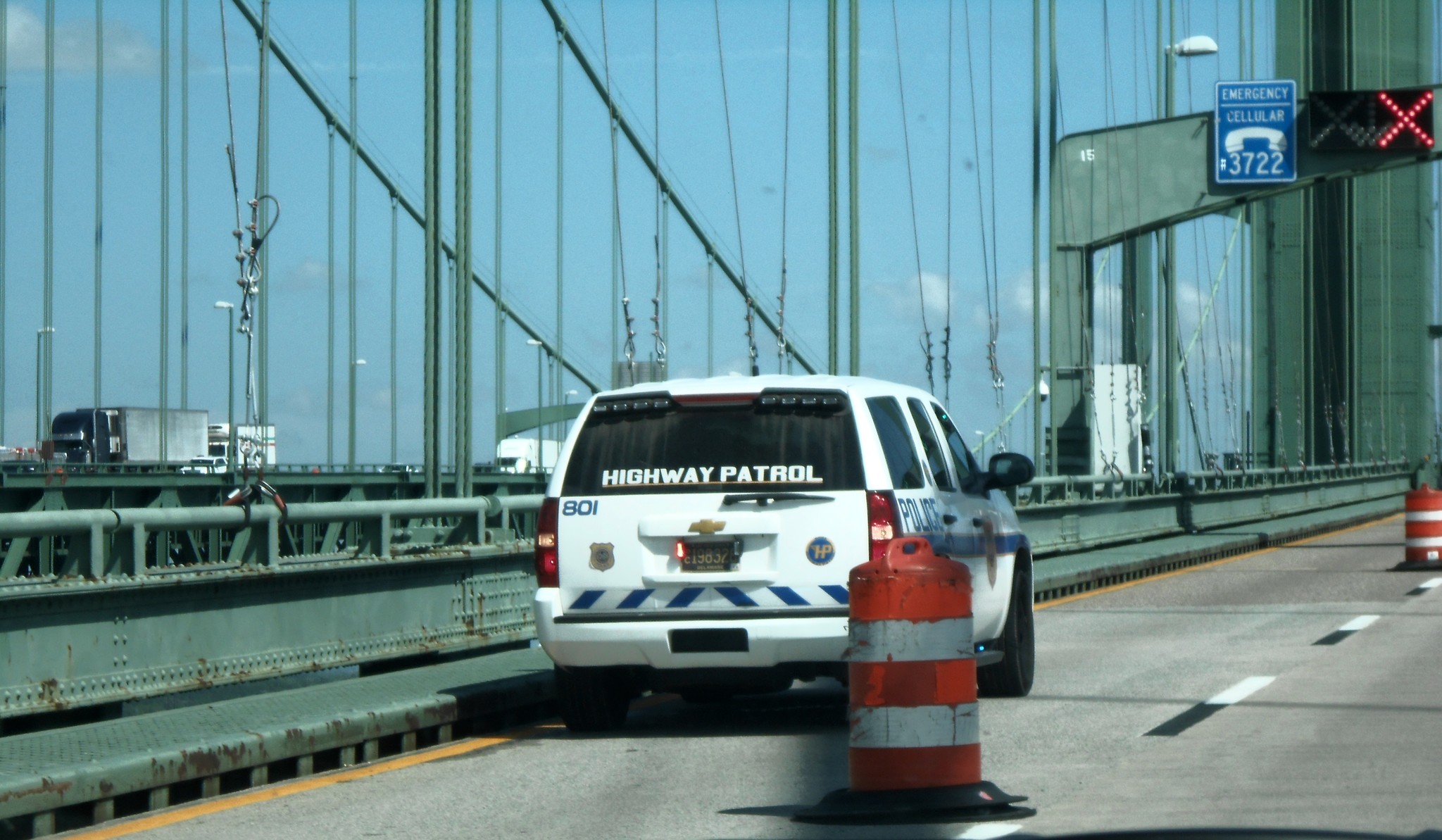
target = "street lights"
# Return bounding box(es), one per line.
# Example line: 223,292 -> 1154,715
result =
1163,36 -> 1220,471
213,301 -> 236,471
35,326 -> 57,450
527,339 -> 544,471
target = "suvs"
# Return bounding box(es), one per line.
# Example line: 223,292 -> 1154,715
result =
533,373 -> 1035,696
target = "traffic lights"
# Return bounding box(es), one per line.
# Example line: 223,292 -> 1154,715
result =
1304,88 -> 1439,156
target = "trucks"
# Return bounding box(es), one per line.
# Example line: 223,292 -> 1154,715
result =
51,406 -> 210,473
181,422 -> 277,475
496,436 -> 565,475
